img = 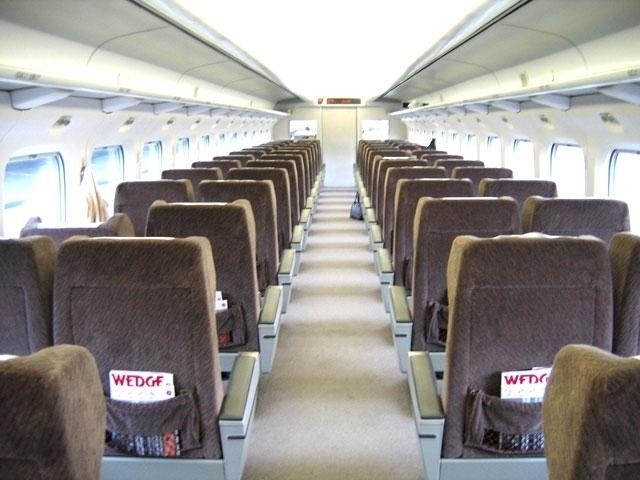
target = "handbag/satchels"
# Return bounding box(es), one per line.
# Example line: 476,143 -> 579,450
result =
350,192 -> 362,220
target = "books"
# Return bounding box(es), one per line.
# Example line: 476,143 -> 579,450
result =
109,371 -> 179,456
215,290 -> 229,346
499,369 -> 551,451
